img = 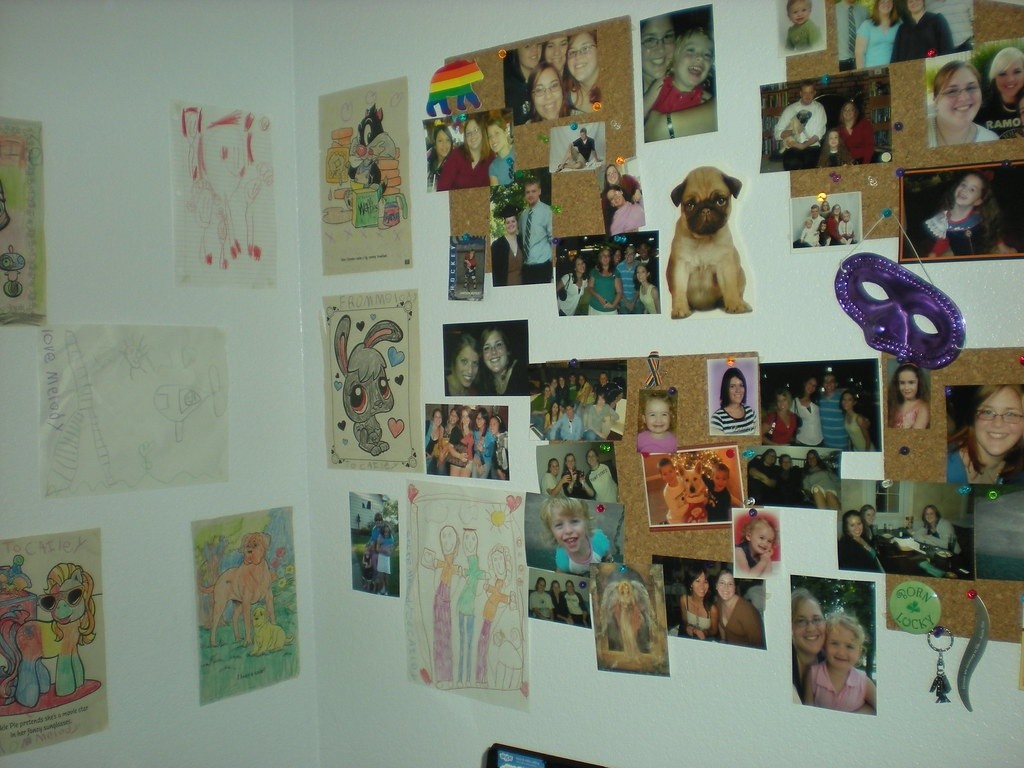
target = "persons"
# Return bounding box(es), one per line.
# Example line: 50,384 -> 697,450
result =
838,504 -> 966,573
791,588 -> 877,715
657,457 -> 742,524
425,326 -> 644,658
426,15 -> 718,316
360,513 -> 394,595
947,384 -> 1024,483
735,516 -> 777,578
637,392 -> 676,453
712,367 -> 877,509
889,362 -> 930,429
774,0 -> 1024,257
679,564 -> 762,646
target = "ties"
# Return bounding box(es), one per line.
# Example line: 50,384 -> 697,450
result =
522,209 -> 533,262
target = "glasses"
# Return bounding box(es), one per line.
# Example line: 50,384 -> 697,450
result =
567,45 -> 597,59
530,83 -> 562,97
465,127 -> 481,137
641,33 -> 677,48
938,82 -> 981,97
795,616 -> 826,626
975,409 -> 1024,423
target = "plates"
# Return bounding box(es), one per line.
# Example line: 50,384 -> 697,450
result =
938,550 -> 953,558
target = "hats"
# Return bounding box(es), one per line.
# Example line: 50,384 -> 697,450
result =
499,205 -> 523,217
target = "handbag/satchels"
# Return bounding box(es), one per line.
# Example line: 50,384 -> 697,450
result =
792,398 -> 803,427
765,412 -> 777,440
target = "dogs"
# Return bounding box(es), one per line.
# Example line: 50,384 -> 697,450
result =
778,109 -> 813,155
665,165 -> 754,320
677,460 -> 716,523
